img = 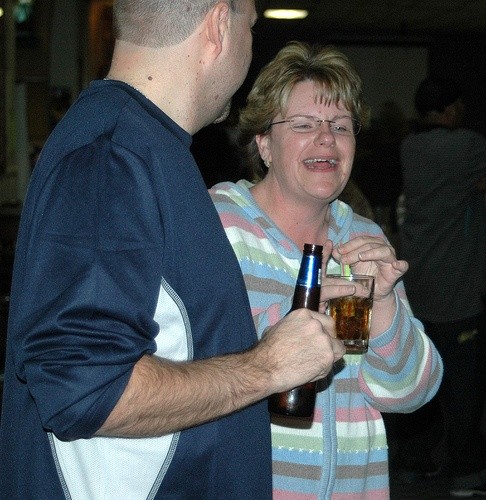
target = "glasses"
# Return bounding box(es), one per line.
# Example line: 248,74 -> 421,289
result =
262,114 -> 362,136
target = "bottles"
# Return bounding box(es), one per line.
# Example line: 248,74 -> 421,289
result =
268,243 -> 323,430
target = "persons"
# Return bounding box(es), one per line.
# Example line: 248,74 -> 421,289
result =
208,41 -> 445,500
396,76 -> 486,500
0,1 -> 346,500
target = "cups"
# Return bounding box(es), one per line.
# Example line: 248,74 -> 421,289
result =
325,274 -> 375,355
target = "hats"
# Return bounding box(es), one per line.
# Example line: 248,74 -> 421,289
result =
415,76 -> 462,113
